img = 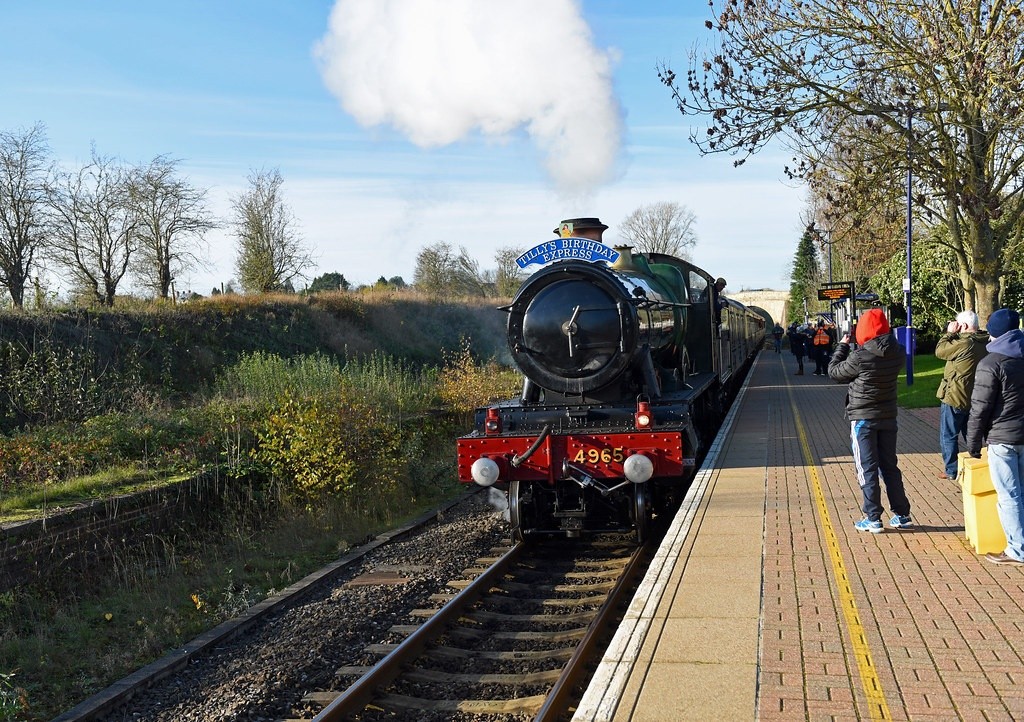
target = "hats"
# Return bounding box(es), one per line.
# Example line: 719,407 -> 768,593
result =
985,309 -> 1020,338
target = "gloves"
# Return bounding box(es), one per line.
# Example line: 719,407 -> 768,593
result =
969,448 -> 983,458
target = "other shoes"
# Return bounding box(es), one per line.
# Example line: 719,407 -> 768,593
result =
775,350 -> 777,352
812,371 -> 820,376
794,373 -> 804,375
779,351 -> 781,353
938,472 -> 956,480
821,373 -> 826,376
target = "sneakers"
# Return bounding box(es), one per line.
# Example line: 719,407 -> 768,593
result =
855,518 -> 885,533
889,514 -> 915,529
984,551 -> 1024,566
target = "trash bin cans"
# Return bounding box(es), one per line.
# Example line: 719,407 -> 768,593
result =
954,446 -> 1009,555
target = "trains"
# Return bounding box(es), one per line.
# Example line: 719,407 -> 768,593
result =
456,218 -> 767,548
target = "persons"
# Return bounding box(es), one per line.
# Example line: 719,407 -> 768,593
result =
827,309 -> 914,533
772,323 -> 784,354
787,321 -> 836,377
936,307 -> 1024,568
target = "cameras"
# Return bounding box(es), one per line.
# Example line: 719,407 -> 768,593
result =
848,336 -> 857,343
